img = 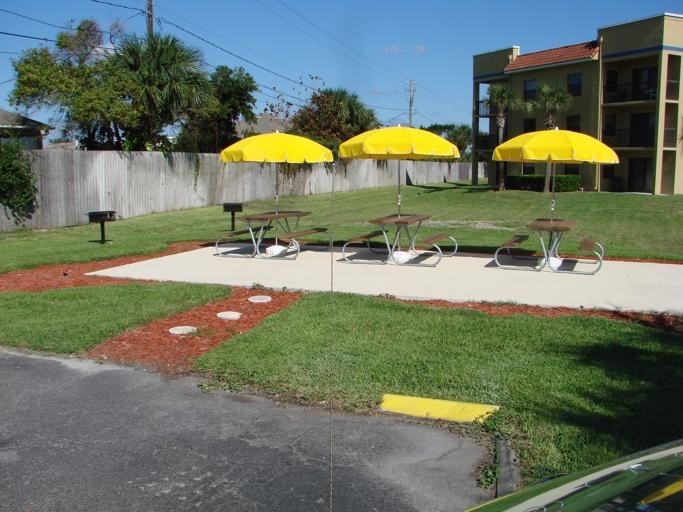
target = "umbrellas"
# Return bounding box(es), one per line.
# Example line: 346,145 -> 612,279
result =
337,122 -> 462,252
491,124 -> 622,255
218,127 -> 335,244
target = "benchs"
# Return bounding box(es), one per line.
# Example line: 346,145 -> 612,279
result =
215,224 -> 272,259
341,229 -> 389,267
269,227 -> 332,261
495,234 -> 529,270
571,237 -> 603,276
417,232 -> 459,268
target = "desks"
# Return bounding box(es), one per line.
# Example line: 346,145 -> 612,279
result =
527,217 -> 571,273
240,209 -> 310,259
367,213 -> 430,266
